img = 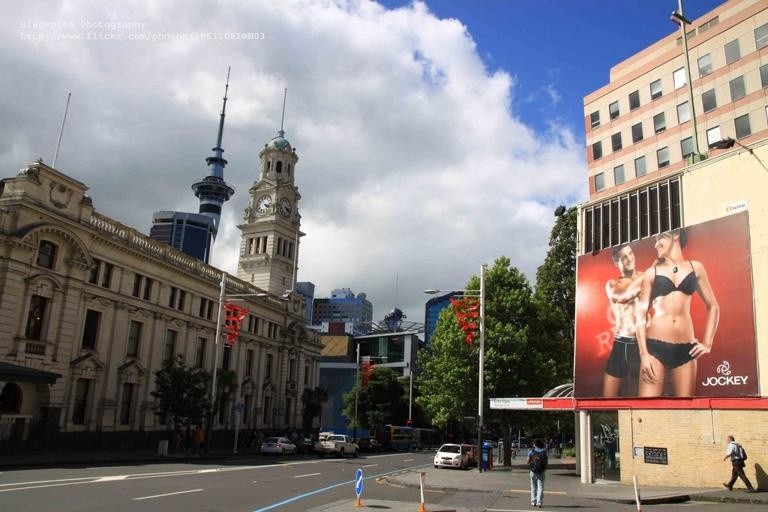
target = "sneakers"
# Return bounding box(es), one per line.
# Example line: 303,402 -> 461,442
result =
744,489 -> 754,493
531,502 -> 541,507
724,484 -> 732,491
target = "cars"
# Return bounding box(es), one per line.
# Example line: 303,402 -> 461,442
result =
261,437 -> 298,456
433,444 -> 468,470
468,437 -> 504,446
460,444 -> 478,464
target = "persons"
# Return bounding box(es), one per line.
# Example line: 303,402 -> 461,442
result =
191,427 -> 204,456
634,226 -> 720,397
601,244 -> 666,398
525,438 -> 549,507
721,435 -> 756,493
511,444 -> 518,459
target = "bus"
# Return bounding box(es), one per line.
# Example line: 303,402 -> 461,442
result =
369,423 -> 413,452
412,428 -> 437,450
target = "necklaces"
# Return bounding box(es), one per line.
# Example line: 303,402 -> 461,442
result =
661,260 -> 686,274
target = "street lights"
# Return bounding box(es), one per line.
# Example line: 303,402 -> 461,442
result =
424,264 -> 486,473
206,273 -> 268,450
354,343 -> 389,425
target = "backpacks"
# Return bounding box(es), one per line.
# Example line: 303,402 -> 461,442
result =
530,449 -> 547,474
732,443 -> 747,460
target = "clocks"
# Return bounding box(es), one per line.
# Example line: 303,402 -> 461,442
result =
277,197 -> 293,218
254,195 -> 273,213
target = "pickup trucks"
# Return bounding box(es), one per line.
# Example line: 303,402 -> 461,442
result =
314,434 -> 360,458
304,432 -> 333,445
